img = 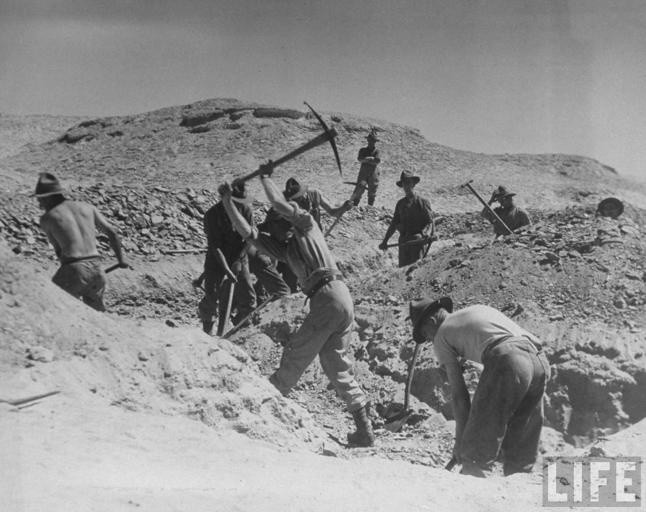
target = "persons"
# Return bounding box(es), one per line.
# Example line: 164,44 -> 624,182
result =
190,174 -> 359,338
408,293 -> 552,479
376,167 -> 438,268
347,128 -> 382,204
218,160 -> 379,448
479,183 -> 531,238
27,171 -> 128,312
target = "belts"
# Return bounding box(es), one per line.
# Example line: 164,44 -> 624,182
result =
308,274 -> 343,298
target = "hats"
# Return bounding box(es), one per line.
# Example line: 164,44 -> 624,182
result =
28,172 -> 67,199
230,178 -> 249,204
494,185 -> 516,201
257,207 -> 290,230
396,168 -> 420,185
410,296 -> 452,344
282,179 -> 308,201
598,197 -> 624,218
364,131 -> 379,143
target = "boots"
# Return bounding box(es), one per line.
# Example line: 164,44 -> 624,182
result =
347,407 -> 376,444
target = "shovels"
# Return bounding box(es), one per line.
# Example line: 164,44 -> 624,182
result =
384,343 -> 424,433
